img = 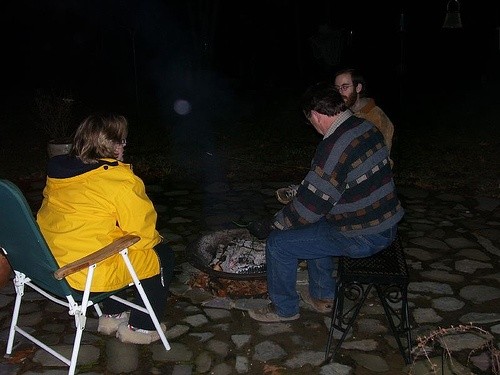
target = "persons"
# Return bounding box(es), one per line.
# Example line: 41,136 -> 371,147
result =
249,83 -> 404,322
276,68 -> 394,273
37,114 -> 174,344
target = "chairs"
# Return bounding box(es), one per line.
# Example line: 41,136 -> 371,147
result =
0,179 -> 172,375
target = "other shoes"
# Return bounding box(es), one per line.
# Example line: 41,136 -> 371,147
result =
97,313 -> 130,336
115,320 -> 167,345
247,303 -> 300,322
300,287 -> 333,314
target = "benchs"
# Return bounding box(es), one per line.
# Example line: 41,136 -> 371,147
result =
326,232 -> 413,364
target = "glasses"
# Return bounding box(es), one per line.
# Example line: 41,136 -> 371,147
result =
336,84 -> 354,91
